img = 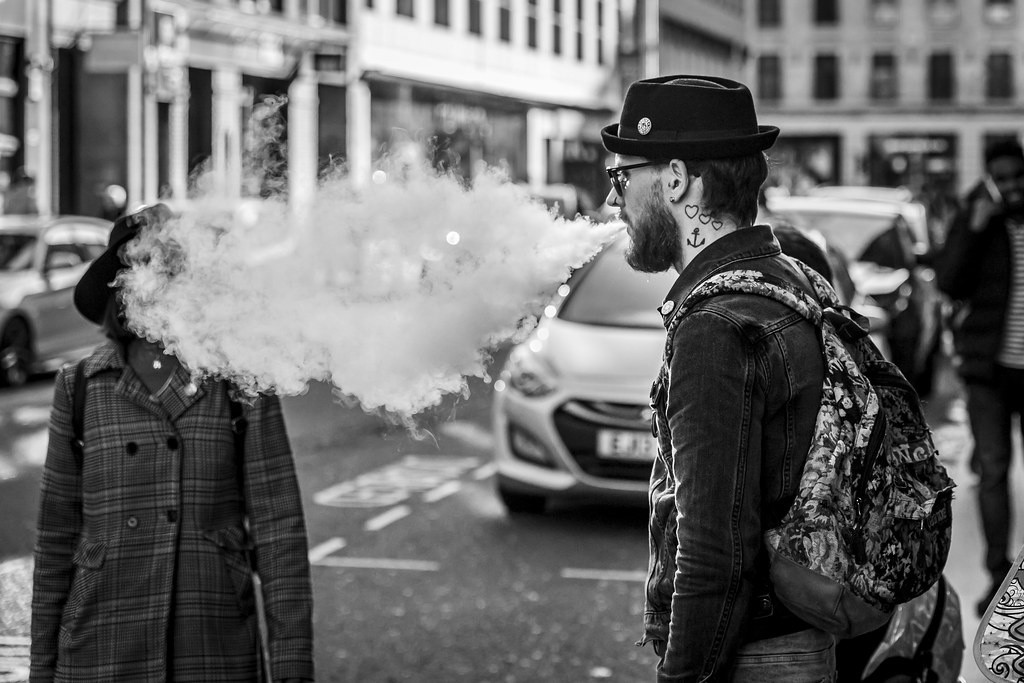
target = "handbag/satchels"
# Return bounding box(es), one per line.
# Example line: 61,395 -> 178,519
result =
863,574 -> 965,681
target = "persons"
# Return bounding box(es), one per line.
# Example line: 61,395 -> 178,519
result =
600,75 -> 898,683
29,203 -> 315,683
939,137 -> 1024,618
751,175 -> 932,397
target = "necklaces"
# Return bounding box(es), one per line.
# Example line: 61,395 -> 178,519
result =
143,341 -> 163,370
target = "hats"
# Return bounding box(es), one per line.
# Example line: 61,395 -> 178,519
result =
73,203 -> 239,330
600,74 -> 781,158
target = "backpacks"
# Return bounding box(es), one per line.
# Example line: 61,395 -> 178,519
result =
649,253 -> 954,605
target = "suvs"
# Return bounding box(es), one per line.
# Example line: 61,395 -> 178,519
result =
757,183 -> 949,397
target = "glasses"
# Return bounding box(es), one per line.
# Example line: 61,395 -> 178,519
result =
605,159 -> 701,197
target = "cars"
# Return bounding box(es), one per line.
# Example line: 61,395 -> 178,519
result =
520,183 -> 594,221
0,213 -> 115,389
491,222 -> 893,528
